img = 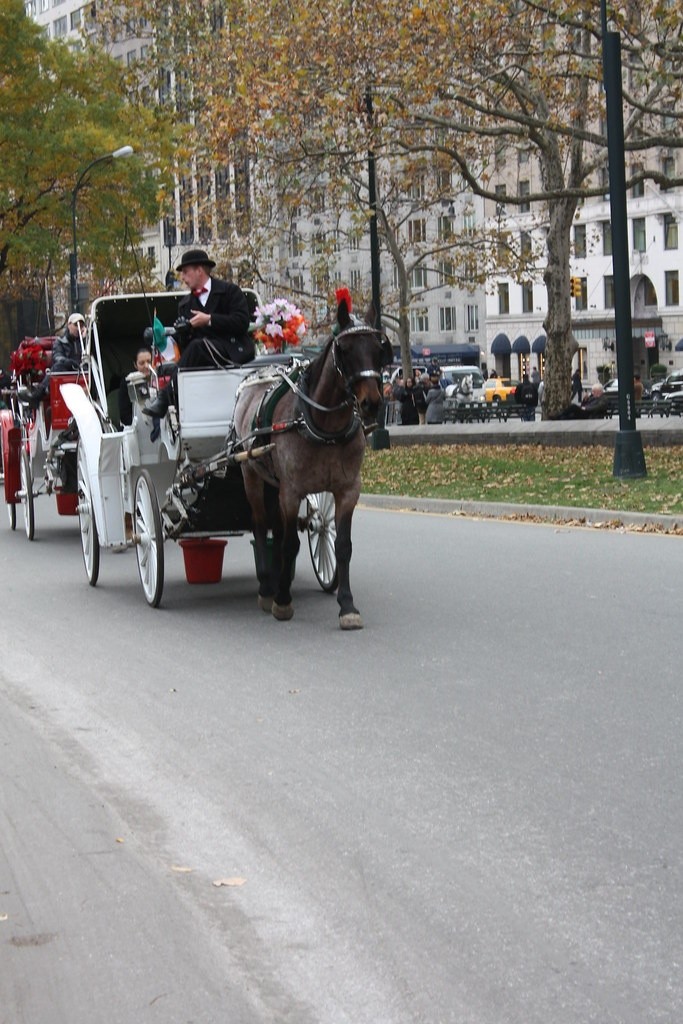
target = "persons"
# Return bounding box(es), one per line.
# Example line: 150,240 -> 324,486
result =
0,313 -> 86,411
581,383 -> 607,419
633,374 -> 643,418
384,357 -> 446,425
571,369 -> 583,402
531,367 -> 540,388
119,249 -> 255,432
483,369 -> 497,381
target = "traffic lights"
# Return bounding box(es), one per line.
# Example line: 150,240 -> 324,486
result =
571,277 -> 582,298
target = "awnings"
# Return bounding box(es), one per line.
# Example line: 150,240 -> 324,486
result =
396,344 -> 480,362
512,336 -> 530,353
675,338 -> 683,351
532,336 -> 546,352
491,333 -> 512,352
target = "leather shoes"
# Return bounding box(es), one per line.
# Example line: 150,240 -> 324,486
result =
141,399 -> 168,419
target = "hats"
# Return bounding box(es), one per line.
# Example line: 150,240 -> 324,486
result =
176,250 -> 216,272
67,313 -> 86,324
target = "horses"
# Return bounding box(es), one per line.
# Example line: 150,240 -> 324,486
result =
446,374 -> 475,423
235,298 -> 386,632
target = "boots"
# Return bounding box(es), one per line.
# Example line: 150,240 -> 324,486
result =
19,388 -> 46,408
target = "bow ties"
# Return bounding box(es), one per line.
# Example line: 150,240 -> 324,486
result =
192,287 -> 209,297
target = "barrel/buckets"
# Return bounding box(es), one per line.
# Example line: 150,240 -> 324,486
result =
180,539 -> 228,584
54,486 -> 79,515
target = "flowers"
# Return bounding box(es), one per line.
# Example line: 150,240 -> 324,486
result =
247,297 -> 309,354
8,337 -> 47,377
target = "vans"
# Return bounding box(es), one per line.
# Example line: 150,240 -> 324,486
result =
437,364 -> 486,406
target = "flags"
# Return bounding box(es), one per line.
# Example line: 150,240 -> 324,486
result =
152,316 -> 180,363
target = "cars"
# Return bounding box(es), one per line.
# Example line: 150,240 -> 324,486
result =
639,369 -> 683,403
480,378 -> 521,404
603,377 -> 618,392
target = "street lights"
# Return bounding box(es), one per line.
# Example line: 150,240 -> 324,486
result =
69,144 -> 133,315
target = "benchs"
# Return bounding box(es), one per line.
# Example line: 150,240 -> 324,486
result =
156,353 -> 290,379
606,400 -> 672,419
442,399 -> 527,425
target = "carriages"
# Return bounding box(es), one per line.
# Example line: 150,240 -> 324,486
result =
0,289 -> 395,632
